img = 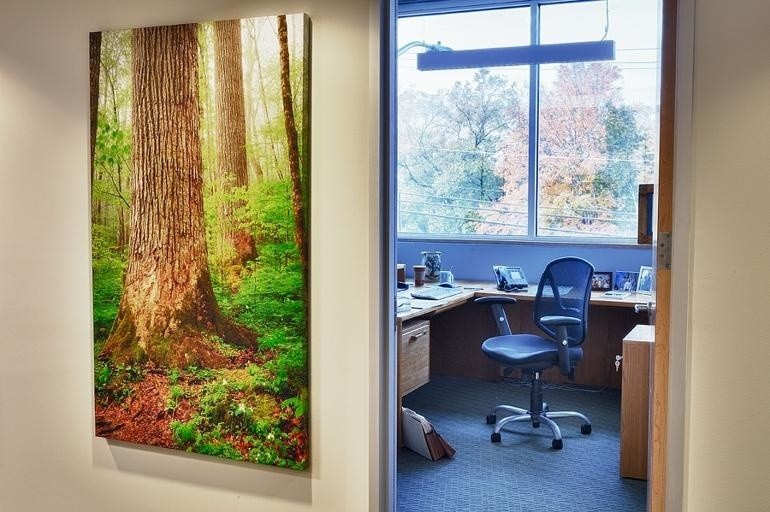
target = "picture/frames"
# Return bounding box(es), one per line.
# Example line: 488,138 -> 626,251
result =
638,183 -> 655,245
591,266 -> 653,296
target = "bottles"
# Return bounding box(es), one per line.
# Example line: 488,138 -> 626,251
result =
420,250 -> 441,282
397,264 -> 405,282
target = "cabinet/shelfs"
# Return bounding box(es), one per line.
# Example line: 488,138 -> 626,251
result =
619,324 -> 655,481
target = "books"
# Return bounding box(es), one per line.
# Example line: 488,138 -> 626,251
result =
429,422 -> 457,457
401,406 -> 445,460
410,286 -> 462,300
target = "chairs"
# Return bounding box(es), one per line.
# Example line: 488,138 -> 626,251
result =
473,256 -> 595,450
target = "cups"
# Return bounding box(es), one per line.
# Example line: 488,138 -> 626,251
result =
412,265 -> 426,286
439,271 -> 454,284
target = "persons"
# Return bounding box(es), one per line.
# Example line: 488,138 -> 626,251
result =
618,276 -> 626,290
623,277 -> 631,292
591,276 -> 610,288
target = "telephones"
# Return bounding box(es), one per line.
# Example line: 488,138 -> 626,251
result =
493,265 -> 529,292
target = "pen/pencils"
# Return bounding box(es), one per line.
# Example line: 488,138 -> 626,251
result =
464,286 -> 483,291
